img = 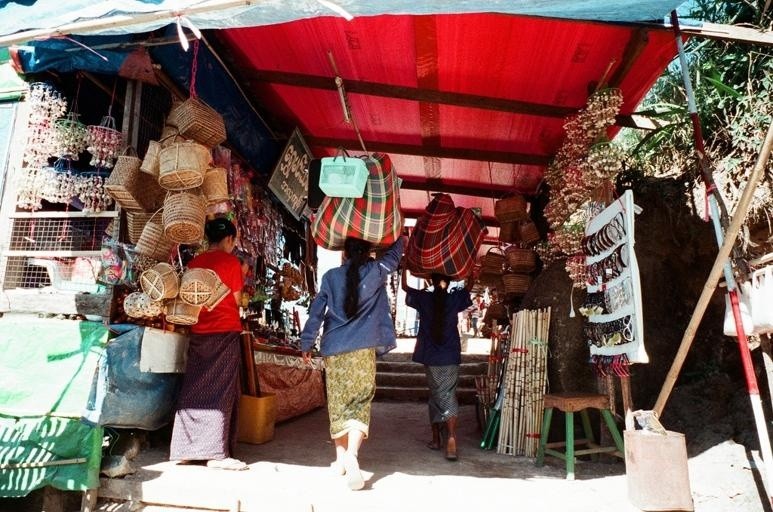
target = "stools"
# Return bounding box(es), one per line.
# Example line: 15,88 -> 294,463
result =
534,394 -> 625,481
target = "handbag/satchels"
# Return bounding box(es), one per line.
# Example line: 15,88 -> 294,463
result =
622,408 -> 696,512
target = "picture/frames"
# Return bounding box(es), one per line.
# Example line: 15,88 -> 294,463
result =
266,125 -> 315,221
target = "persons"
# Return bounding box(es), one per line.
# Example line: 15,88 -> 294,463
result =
298,237 -> 409,490
401,258 -> 477,461
167,218 -> 249,471
